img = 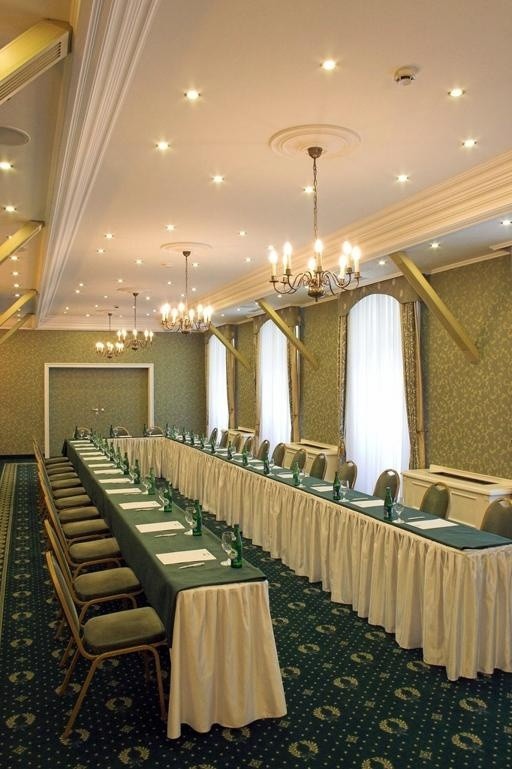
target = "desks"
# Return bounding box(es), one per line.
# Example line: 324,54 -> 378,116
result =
62,438 -> 271,741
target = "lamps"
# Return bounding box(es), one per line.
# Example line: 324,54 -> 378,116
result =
160,250 -> 214,337
93,313 -> 123,358
265,146 -> 368,304
116,292 -> 154,349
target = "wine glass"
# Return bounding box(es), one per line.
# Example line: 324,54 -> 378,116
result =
220,529 -> 234,571
392,496 -> 409,526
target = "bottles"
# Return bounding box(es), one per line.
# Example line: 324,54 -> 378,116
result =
383,485 -> 394,521
89,422 -> 349,538
230,522 -> 245,567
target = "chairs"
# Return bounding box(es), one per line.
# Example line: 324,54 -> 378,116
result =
44,550 -> 167,740
42,519 -> 143,668
32,425 -> 169,621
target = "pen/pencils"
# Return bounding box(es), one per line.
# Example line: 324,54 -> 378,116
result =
154,534 -> 176,537
179,563 -> 205,568
353,498 -> 368,500
137,509 -> 153,511
408,517 -> 424,519
314,484 -> 326,485
